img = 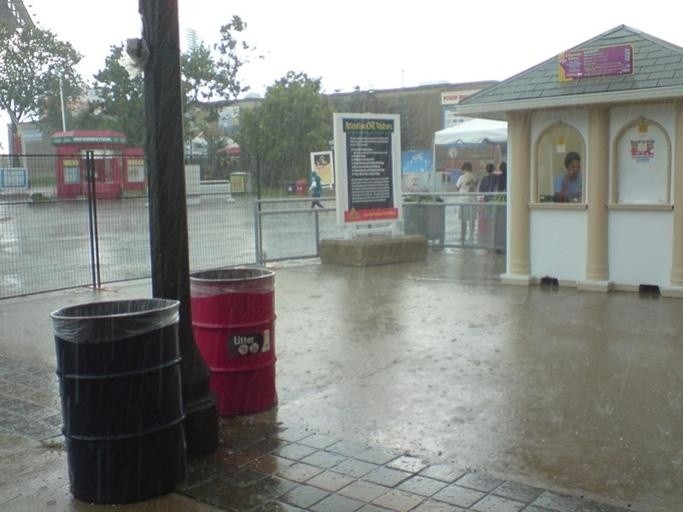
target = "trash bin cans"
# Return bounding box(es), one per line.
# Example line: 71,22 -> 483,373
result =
48,298 -> 186,505
287,182 -> 307,196
403,204 -> 445,244
190,267 -> 279,416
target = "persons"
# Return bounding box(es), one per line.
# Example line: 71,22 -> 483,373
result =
478,162 -> 501,202
552,152 -> 583,202
497,161 -> 507,202
455,161 -> 479,242
306,170 -> 329,216
